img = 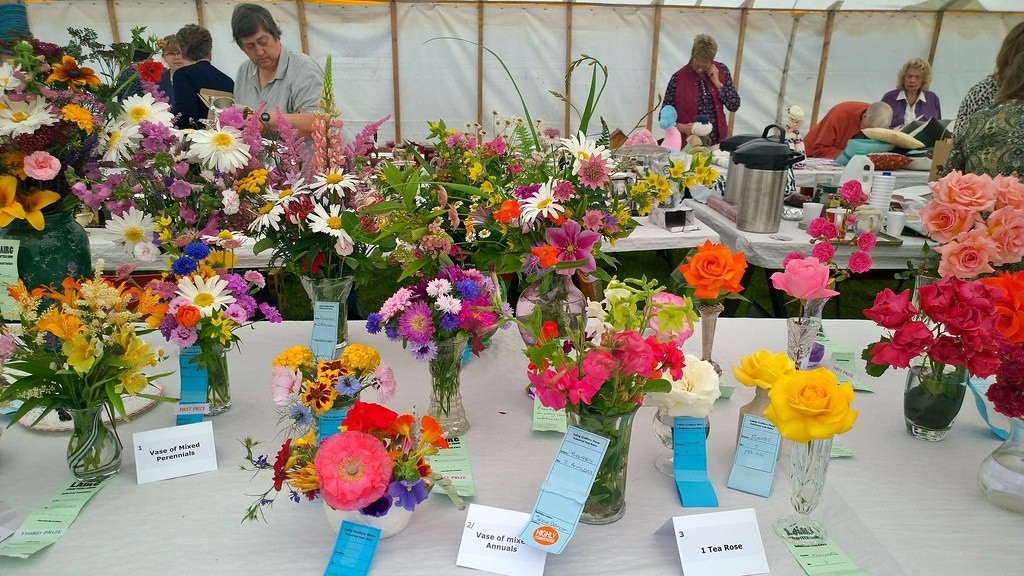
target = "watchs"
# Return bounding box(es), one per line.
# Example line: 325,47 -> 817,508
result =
261,112 -> 270,130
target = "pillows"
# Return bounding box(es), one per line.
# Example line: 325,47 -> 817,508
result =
900,120 -> 952,135
860,128 -> 926,152
910,116 -> 954,146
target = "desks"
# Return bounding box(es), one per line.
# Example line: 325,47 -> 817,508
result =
0,165 -> 720,313
686,167 -> 1024,289
0,314 -> 1024,576
717,153 -> 931,188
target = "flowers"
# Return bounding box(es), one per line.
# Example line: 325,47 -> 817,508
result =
0,26 -> 1024,526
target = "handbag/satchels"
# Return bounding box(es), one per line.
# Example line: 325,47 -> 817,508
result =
928,138 -> 954,185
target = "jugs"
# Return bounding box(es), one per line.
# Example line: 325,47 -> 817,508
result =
718,133 -> 762,208
733,123 -> 805,235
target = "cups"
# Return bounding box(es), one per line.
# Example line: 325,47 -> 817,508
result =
855,209 -> 882,237
885,211 -> 909,237
826,208 -> 848,239
867,174 -> 896,236
803,202 -> 825,225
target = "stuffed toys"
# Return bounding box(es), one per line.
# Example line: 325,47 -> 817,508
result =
784,105 -> 806,164
684,115 -> 713,149
660,105 -> 681,152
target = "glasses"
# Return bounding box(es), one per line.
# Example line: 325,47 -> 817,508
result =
691,61 -> 709,71
162,50 -> 182,57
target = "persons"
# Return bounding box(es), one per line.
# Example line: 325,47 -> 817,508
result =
231,4 -> 357,178
881,57 -> 941,129
944,22 -> 1024,219
173,24 -> 234,177
117,34 -> 188,106
801,101 -> 893,197
659,32 -> 740,150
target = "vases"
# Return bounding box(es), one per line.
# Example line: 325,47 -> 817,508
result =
0,221 -> 1024,539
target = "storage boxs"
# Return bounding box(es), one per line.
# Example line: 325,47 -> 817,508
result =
196,88 -> 238,129
650,204 -> 701,233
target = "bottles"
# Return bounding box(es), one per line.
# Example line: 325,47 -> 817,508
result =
837,150 -> 875,203
